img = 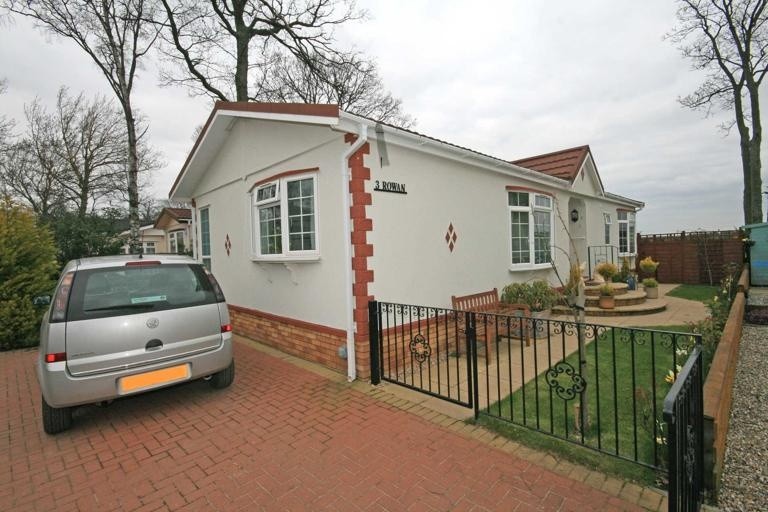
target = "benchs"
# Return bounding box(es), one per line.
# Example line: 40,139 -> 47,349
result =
451,288 -> 531,365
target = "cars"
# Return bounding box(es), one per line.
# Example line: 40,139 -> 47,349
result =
33,253 -> 234,434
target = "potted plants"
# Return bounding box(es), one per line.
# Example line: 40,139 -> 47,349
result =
597,263 -> 619,309
640,256 -> 660,299
